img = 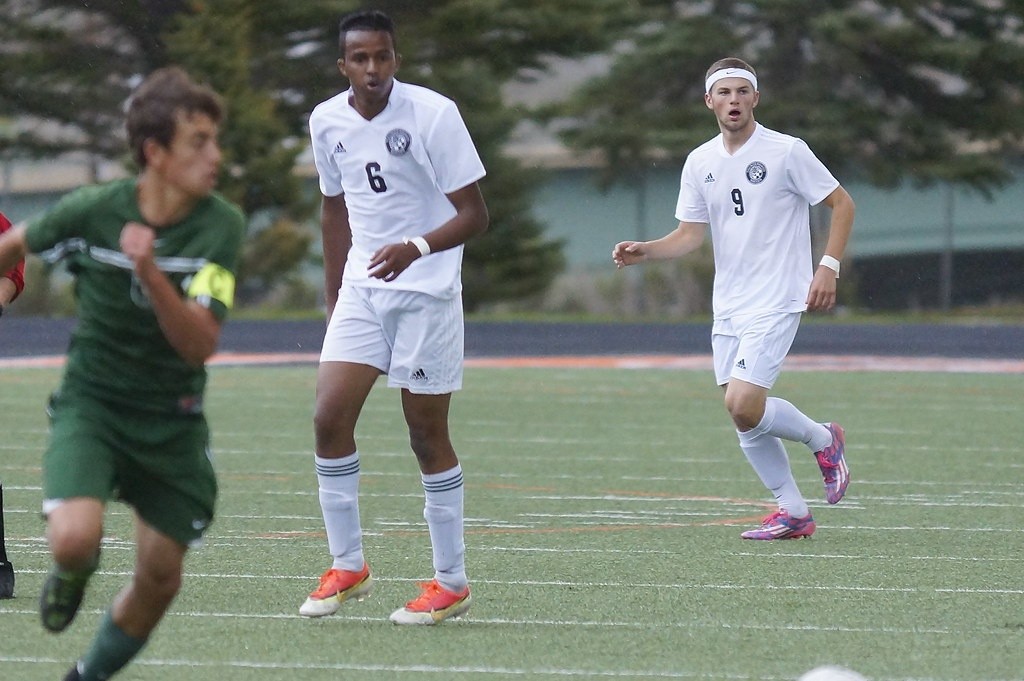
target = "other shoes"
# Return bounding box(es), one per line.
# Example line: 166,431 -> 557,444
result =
0,561 -> 15,598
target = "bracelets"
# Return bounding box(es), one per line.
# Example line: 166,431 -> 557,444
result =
819,255 -> 841,273
409,236 -> 430,256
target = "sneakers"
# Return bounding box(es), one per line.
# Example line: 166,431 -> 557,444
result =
741,512 -> 816,541
40,548 -> 100,632
813,422 -> 851,504
299,562 -> 373,618
389,578 -> 472,627
64,668 -> 81,681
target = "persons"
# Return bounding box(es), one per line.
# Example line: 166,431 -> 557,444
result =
299,12 -> 489,625
0,65 -> 246,681
612,58 -> 849,539
